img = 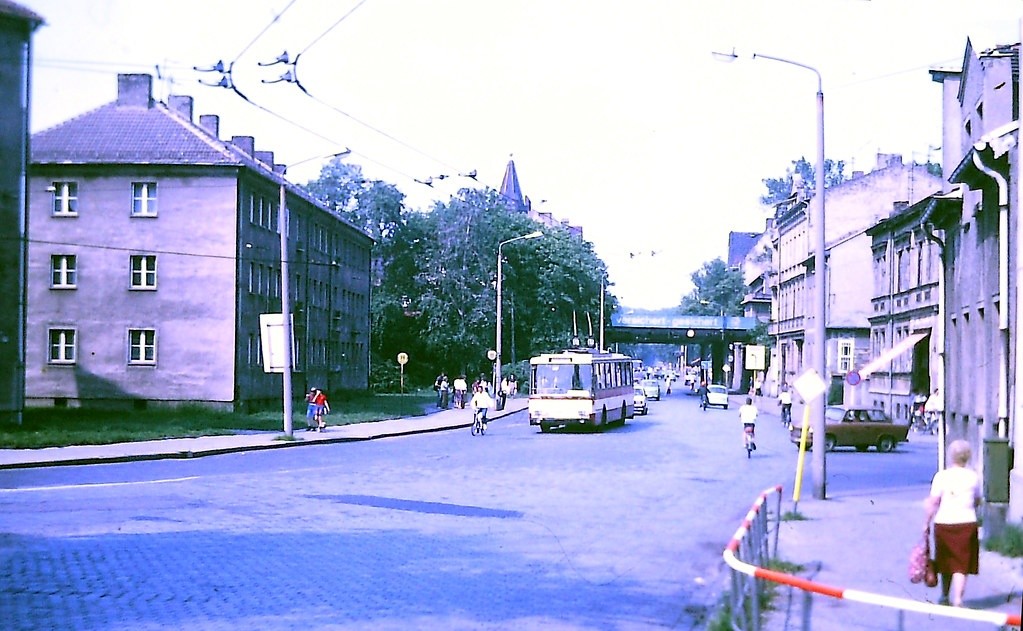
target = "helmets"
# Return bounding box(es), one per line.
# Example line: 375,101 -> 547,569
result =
783,387 -> 788,391
701,382 -> 705,386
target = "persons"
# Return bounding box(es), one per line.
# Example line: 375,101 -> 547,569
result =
739,398 -> 756,451
304,387 -> 331,433
435,372 -> 518,434
777,386 -> 793,427
663,371 -> 763,412
906,388 -> 939,433
923,439 -> 983,608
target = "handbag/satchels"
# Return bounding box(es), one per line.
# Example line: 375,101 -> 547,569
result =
908,534 -> 929,583
323,408 -> 328,415
925,537 -> 938,587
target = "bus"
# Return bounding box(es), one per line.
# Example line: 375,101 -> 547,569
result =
632,360 -> 642,382
522,347 -> 636,433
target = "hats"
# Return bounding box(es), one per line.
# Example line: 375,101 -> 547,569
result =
746,398 -> 751,404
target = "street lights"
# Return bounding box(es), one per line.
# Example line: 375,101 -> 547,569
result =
709,46 -> 829,500
495,231 -> 544,395
279,147 -> 352,437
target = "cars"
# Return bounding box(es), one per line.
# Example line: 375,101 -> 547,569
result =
790,405 -> 911,454
706,385 -> 729,409
633,369 -> 678,415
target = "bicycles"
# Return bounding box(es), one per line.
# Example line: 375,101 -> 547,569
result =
472,409 -> 488,437
911,410 -> 940,435
739,414 -> 758,459
697,391 -> 711,410
778,404 -> 794,428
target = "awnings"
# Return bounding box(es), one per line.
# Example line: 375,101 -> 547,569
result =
859,335 -> 926,377
